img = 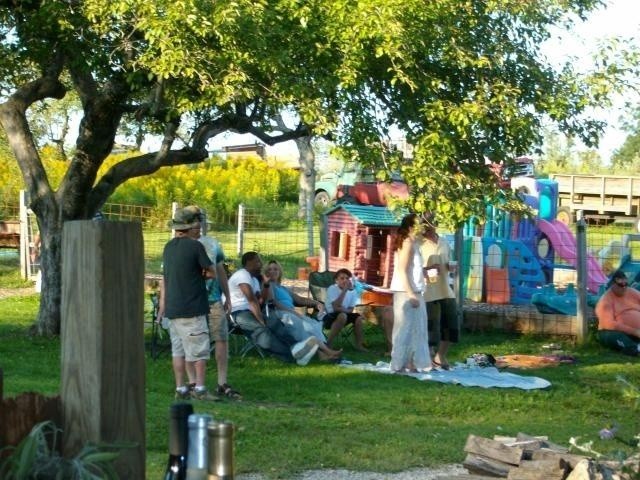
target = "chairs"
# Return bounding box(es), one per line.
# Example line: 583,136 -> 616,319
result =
148,291 -> 171,359
208,308 -> 264,363
307,270 -> 355,348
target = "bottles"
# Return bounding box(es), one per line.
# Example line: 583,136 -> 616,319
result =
185,415 -> 214,480
163,402 -> 193,480
206,422 -> 233,480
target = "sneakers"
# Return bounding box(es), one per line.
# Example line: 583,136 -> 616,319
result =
296,344 -> 319,366
175,390 -> 191,399
192,389 -> 221,401
291,336 -> 317,359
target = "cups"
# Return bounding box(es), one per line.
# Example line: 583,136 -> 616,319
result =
427,269 -> 438,284
448,261 -> 458,273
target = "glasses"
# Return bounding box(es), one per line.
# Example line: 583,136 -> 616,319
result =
613,279 -> 627,287
194,213 -> 206,223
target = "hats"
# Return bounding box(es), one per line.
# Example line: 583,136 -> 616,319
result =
168,208 -> 194,230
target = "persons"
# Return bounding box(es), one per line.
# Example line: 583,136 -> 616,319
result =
188,205 -> 241,400
223,252 -> 368,366
391,215 -> 432,372
154,207 -> 220,400
418,212 -> 457,372
595,273 -> 640,357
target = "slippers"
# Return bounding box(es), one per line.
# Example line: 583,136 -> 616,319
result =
432,359 -> 454,370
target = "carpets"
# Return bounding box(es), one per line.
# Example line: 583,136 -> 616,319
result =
490,351 -> 581,370
335,358 -> 553,391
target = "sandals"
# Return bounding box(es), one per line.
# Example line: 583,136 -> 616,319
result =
217,383 -> 242,400
